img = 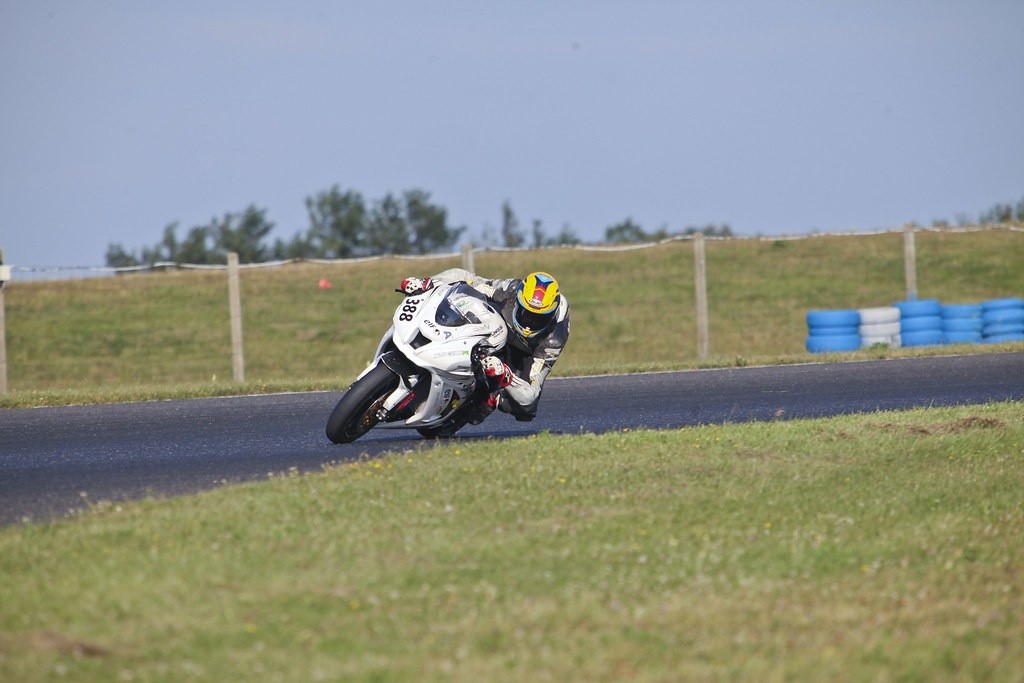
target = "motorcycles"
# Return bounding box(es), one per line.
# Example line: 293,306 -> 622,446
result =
325,276 -> 511,444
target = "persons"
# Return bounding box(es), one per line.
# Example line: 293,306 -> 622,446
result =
400,267 -> 571,423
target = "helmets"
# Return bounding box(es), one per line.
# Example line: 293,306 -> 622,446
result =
511,271 -> 560,340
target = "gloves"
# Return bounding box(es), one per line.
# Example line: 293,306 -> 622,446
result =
482,355 -> 512,386
401,274 -> 434,296
